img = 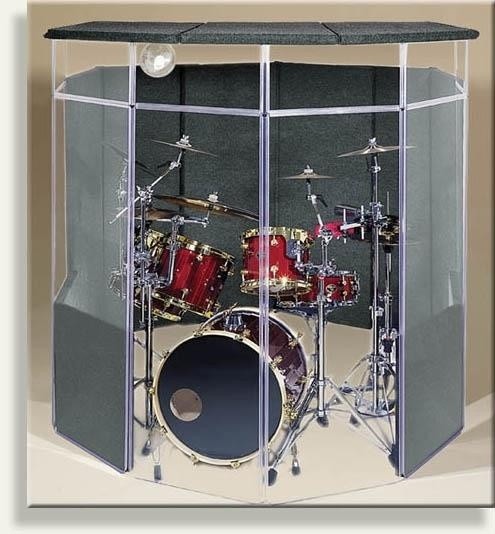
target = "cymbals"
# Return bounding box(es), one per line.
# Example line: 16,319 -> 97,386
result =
152,194 -> 258,222
280,168 -> 332,180
151,137 -> 216,158
337,139 -> 414,157
102,142 -> 155,176
107,207 -> 183,222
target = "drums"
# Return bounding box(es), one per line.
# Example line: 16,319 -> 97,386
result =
107,224 -> 187,322
240,228 -> 312,297
146,233 -> 236,319
153,305 -> 309,469
274,265 -> 360,308
